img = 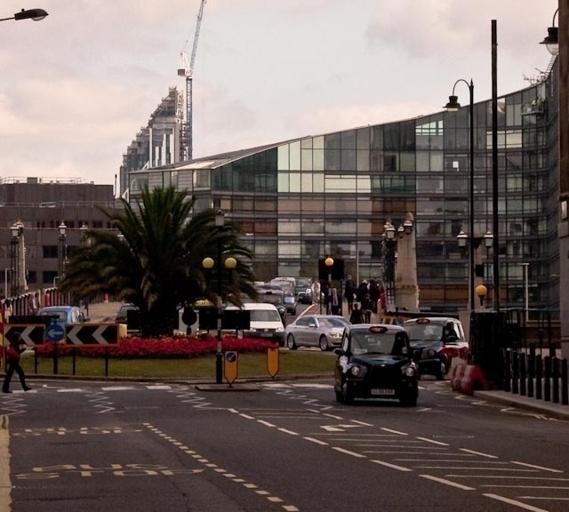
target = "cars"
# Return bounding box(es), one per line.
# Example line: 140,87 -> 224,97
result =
35,306 -> 80,323
334,316 -> 469,407
241,303 -> 284,346
254,277 -> 313,314
286,315 -> 352,351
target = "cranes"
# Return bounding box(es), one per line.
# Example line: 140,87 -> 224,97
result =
178,0 -> 207,160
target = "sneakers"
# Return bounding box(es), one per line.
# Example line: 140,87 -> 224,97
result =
1,386 -> 32,394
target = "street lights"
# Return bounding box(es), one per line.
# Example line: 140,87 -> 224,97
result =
539,7 -> 560,55
381,221 -> 395,307
57,219 -> 67,278
11,223 -> 22,296
324,257 -> 335,283
202,257 -> 237,384
475,285 -> 488,307
443,77 -> 475,319
0,9 -> 49,22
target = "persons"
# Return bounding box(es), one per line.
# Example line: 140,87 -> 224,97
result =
318,271 -> 386,325
2,332 -> 31,393
